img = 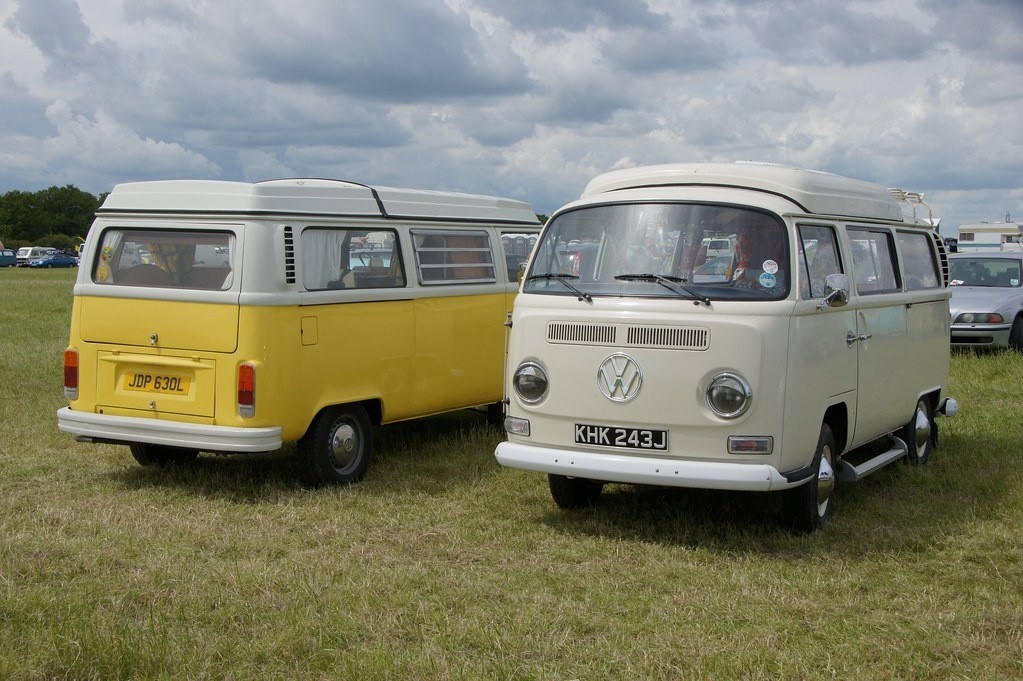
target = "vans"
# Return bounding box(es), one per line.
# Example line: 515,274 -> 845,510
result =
706,238 -> 736,265
56,179 -> 552,494
77,243 -> 139,271
497,162 -> 958,535
0,249 -> 17,268
15,246 -> 57,268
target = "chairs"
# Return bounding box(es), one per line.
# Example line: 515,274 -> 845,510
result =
118,263 -> 175,285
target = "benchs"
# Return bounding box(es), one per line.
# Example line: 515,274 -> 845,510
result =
179,266 -> 230,288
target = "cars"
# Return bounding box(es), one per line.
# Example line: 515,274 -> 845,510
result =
693,255 -> 733,282
29,253 -> 77,269
555,242 -> 656,281
926,251 -> 1023,354
